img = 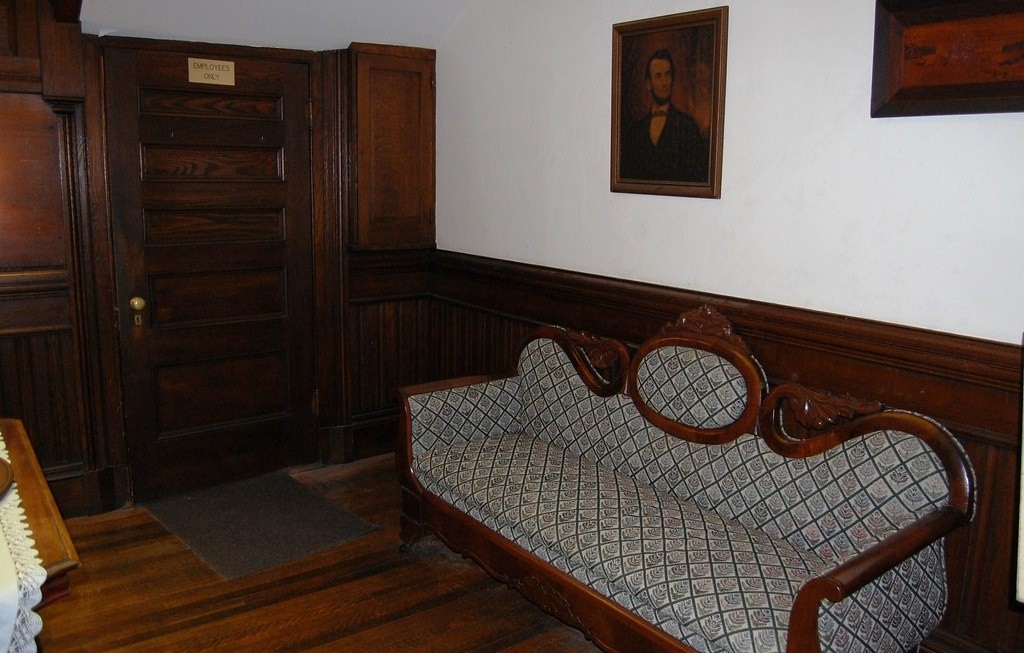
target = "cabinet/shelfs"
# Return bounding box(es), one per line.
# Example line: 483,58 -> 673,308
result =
342,41 -> 438,255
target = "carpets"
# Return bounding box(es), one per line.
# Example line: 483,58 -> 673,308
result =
136,475 -> 382,580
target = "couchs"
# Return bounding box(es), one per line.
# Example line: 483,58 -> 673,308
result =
398,306 -> 982,653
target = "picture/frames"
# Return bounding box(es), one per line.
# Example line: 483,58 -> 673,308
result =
609,5 -> 729,198
870,0 -> 1023,117
